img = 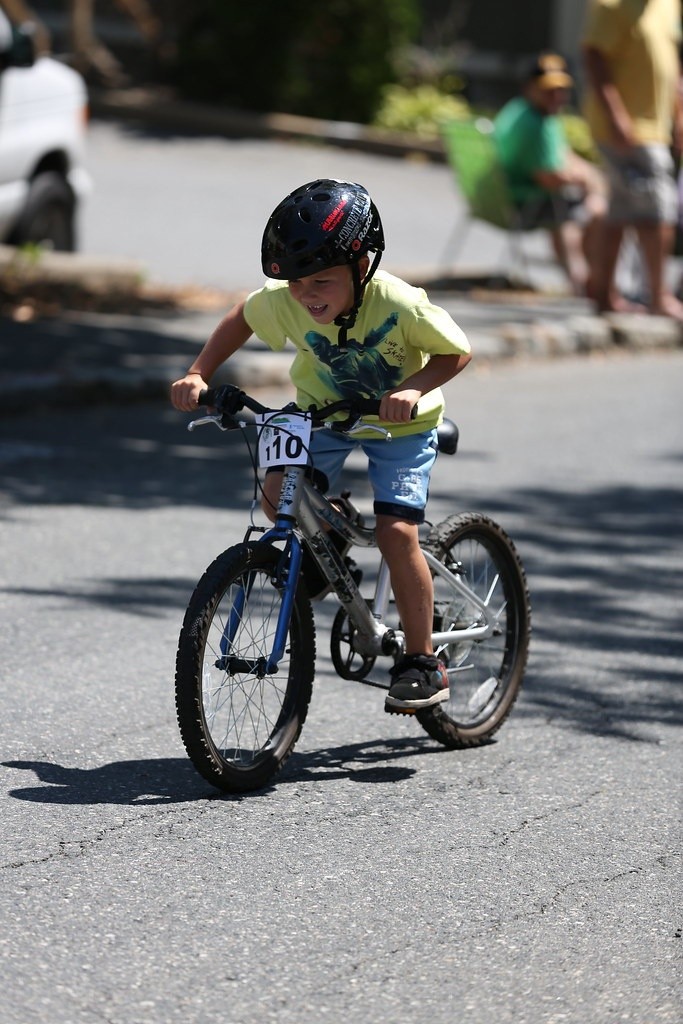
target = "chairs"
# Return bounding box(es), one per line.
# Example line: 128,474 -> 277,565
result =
441,119 -> 569,292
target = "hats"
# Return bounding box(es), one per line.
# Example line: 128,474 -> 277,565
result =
528,51 -> 574,90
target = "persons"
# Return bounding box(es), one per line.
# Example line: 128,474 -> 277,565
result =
572,2 -> 682,320
499,54 -> 642,313
171,179 -> 480,711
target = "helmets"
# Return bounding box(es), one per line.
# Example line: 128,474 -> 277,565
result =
262,179 -> 386,278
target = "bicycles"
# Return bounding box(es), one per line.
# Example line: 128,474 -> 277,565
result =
173,382 -> 531,791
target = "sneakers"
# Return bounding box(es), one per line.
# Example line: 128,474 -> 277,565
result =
298,528 -> 351,604
387,653 -> 450,708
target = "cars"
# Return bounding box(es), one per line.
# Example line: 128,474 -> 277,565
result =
0,3 -> 87,253
